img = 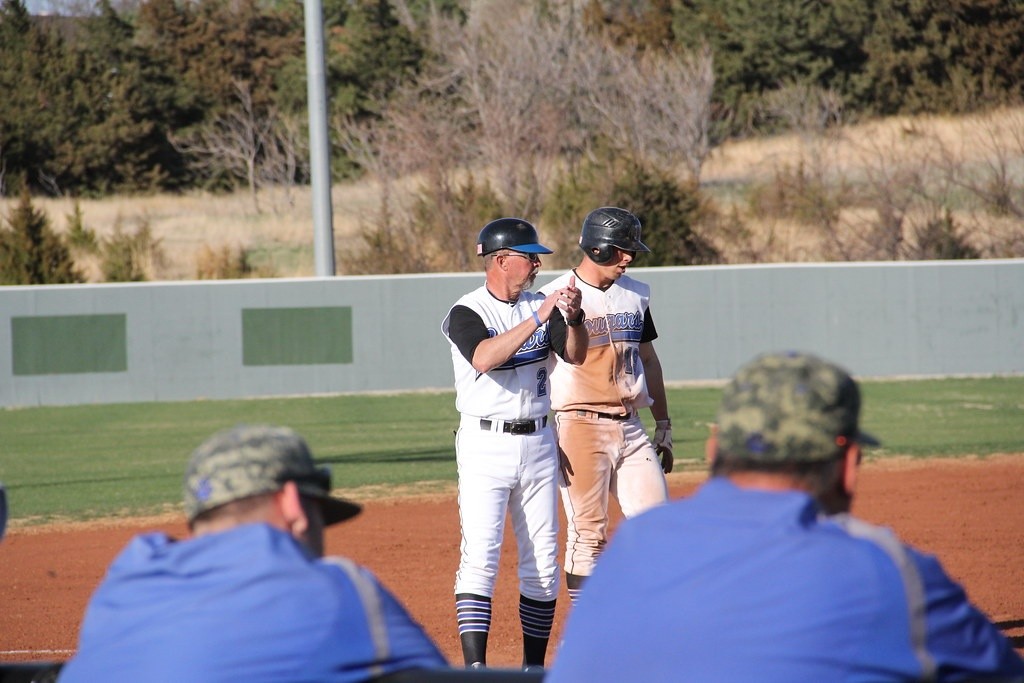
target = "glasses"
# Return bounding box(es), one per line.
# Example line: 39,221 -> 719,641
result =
275,467 -> 332,489
492,252 -> 537,261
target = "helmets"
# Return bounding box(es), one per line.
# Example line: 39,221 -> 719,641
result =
577,207 -> 651,264
476,218 -> 553,256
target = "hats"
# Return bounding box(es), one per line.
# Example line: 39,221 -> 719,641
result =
180,426 -> 362,527
716,352 -> 882,461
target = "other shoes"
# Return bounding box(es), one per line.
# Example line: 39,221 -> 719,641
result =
471,662 -> 487,671
523,665 -> 544,674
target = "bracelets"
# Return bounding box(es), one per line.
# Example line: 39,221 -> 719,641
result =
534,311 -> 542,327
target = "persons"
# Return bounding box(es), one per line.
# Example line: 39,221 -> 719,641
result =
546,352 -> 1024,683
62,427 -> 449,683
441,218 -> 589,671
534,208 -> 674,602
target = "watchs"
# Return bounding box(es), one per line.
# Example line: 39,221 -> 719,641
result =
566,308 -> 586,327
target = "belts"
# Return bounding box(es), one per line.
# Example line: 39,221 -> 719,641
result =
578,411 -> 631,420
480,415 -> 547,435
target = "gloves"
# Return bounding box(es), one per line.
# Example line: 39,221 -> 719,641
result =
652,419 -> 674,474
